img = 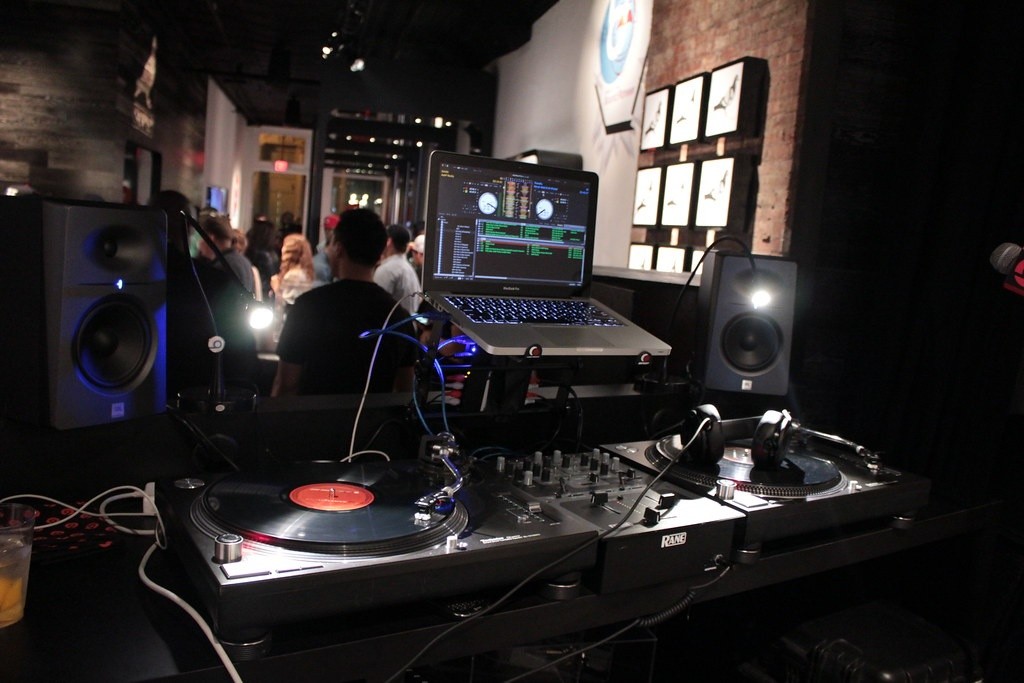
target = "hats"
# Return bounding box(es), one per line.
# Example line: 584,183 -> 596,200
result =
324,215 -> 340,230
408,235 -> 424,254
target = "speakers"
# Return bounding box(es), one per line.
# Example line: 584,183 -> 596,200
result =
0,196 -> 167,431
692,249 -> 798,397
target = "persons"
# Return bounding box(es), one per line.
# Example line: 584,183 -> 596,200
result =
149,190 -> 429,399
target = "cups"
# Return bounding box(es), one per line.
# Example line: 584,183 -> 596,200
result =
0,503 -> 36,628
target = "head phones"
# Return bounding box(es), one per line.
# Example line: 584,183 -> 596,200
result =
681,404 -> 792,470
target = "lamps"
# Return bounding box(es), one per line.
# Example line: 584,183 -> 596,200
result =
668,235 -> 770,344
178,208 -> 273,395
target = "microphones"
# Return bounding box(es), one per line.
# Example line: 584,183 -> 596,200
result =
989,243 -> 1024,296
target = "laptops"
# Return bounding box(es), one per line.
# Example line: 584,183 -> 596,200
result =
419,150 -> 673,356
